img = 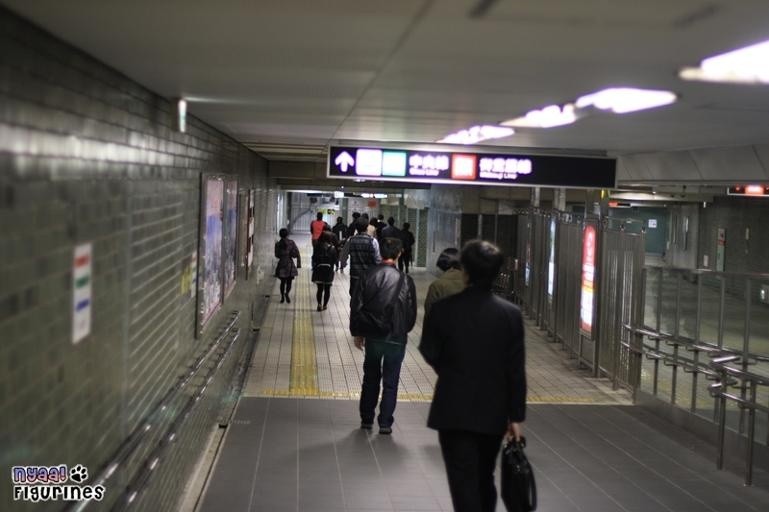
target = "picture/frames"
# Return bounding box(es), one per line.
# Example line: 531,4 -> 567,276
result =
195,174 -> 257,343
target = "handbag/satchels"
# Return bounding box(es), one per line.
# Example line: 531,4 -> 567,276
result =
501,444 -> 537,512
290,247 -> 299,258
359,308 -> 397,339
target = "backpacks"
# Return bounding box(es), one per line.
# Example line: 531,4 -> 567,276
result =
316,244 -> 333,277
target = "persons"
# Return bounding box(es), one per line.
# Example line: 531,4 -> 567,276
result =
423,247 -> 468,316
415,237 -> 529,512
348,236 -> 419,435
273,212 -> 415,312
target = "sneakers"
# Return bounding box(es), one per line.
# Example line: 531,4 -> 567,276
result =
379,423 -> 392,434
318,303 -> 327,311
362,418 -> 373,428
281,292 -> 290,303
